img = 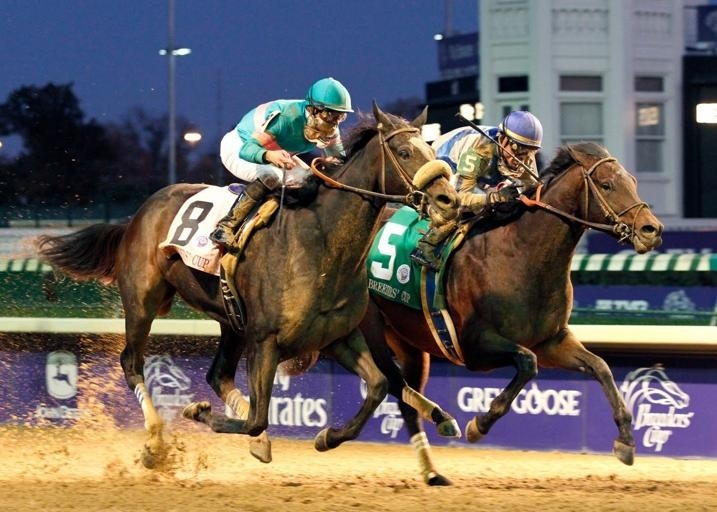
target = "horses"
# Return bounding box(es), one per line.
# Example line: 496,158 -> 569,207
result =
280,142 -> 665,486
33,97 -> 462,469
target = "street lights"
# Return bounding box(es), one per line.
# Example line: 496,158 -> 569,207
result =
159,45 -> 193,187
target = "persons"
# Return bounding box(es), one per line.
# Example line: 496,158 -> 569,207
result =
207,77 -> 355,253
410,110 -> 544,272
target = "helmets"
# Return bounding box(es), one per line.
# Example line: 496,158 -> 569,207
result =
306,77 -> 354,113
497,111 -> 543,147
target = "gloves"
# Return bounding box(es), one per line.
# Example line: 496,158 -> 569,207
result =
486,181 -> 524,206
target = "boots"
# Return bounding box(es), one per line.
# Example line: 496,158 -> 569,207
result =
411,218 -> 457,272
209,189 -> 258,252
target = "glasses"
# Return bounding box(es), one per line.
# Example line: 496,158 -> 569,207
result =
509,139 -> 537,157
314,108 -> 345,125
